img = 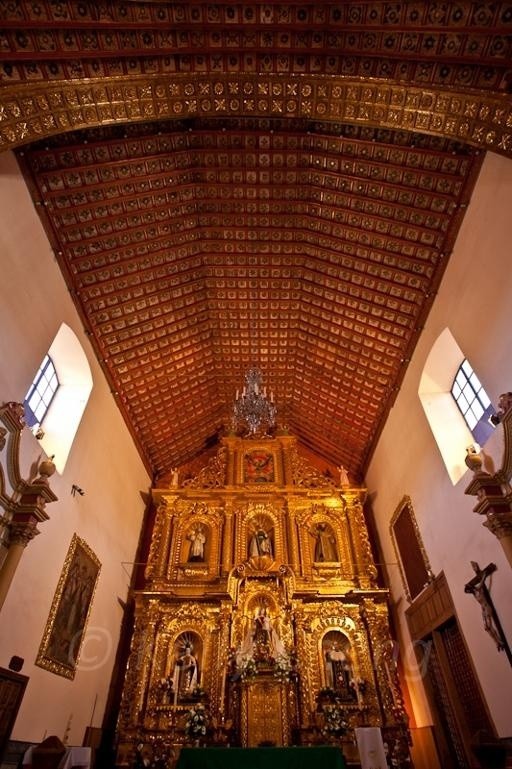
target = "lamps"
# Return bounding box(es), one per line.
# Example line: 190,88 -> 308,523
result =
232,370 -> 277,436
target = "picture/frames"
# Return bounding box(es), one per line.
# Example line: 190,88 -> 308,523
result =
35,532 -> 102,681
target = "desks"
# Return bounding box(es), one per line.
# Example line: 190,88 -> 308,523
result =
22,745 -> 92,769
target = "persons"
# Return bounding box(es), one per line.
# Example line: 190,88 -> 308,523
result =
307,523 -> 338,562
337,465 -> 349,485
326,641 -> 351,686
171,467 -> 180,486
188,527 -> 206,560
180,642 -> 198,693
249,524 -> 272,560
250,605 -> 271,641
